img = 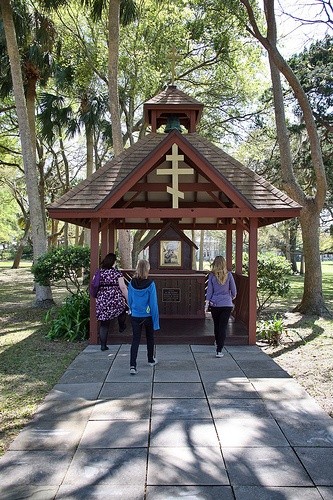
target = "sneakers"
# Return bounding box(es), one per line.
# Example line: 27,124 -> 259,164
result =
215,352 -> 224,357
214,340 -> 218,347
148,360 -> 157,366
130,366 -> 138,373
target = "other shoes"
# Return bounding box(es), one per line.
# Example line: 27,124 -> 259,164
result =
120,325 -> 126,332
100,346 -> 109,350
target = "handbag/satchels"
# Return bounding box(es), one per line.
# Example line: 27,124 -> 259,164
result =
90,268 -> 100,297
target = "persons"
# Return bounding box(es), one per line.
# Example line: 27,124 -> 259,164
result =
206,256 -> 237,359
127,259 -> 160,374
93,253 -> 130,351
